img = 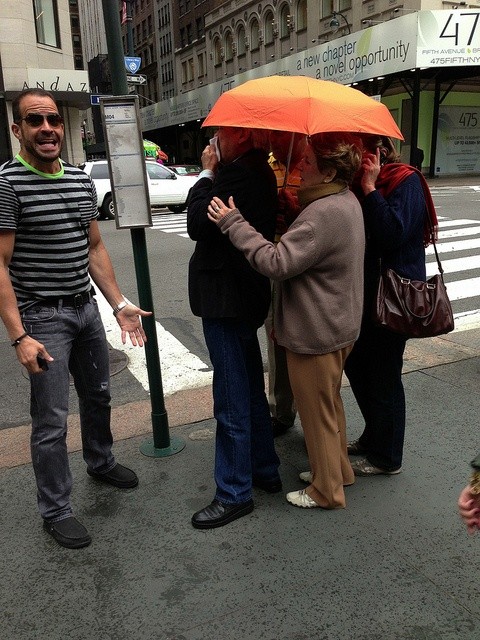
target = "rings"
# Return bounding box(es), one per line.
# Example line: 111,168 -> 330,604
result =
214,207 -> 219,211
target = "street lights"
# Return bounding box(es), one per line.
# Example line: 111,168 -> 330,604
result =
81,121 -> 88,162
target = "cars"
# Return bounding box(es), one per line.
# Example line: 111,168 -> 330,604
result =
167,165 -> 202,176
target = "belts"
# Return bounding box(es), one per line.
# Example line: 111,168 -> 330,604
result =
39,286 -> 96,308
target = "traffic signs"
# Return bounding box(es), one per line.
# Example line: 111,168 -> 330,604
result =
126,73 -> 148,85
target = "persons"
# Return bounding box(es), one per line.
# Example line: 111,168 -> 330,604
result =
458,449 -> 480,530
345,138 -> 431,478
186,125 -> 281,528
0,89 -> 153,548
265,130 -> 313,458
206,131 -> 367,510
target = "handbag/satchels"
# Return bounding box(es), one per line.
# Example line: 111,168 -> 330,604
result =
374,182 -> 456,337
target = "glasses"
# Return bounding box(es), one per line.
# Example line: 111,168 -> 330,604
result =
17,113 -> 65,128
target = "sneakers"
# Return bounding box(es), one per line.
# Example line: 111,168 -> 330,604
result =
350,458 -> 401,477
347,437 -> 365,455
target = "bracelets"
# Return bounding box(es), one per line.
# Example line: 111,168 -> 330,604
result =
11,332 -> 28,347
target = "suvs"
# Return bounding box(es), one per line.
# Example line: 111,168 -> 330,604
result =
79,160 -> 199,219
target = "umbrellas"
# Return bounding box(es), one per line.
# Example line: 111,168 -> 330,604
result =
201,75 -> 406,189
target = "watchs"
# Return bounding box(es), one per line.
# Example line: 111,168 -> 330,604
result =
113,301 -> 127,316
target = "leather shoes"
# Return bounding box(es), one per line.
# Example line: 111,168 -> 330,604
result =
285,489 -> 320,509
191,496 -> 254,529
299,471 -> 314,484
252,480 -> 282,492
86,463 -> 138,488
43,517 -> 90,549
